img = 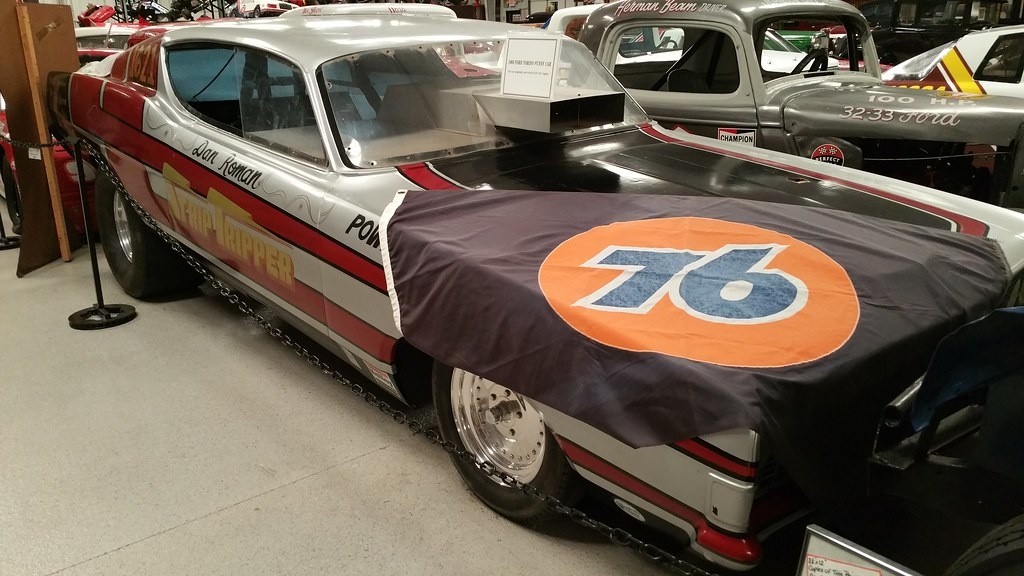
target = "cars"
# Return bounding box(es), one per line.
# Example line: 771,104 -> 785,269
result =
45,0 -> 1024,573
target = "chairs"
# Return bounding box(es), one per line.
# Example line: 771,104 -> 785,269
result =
668,69 -> 711,94
